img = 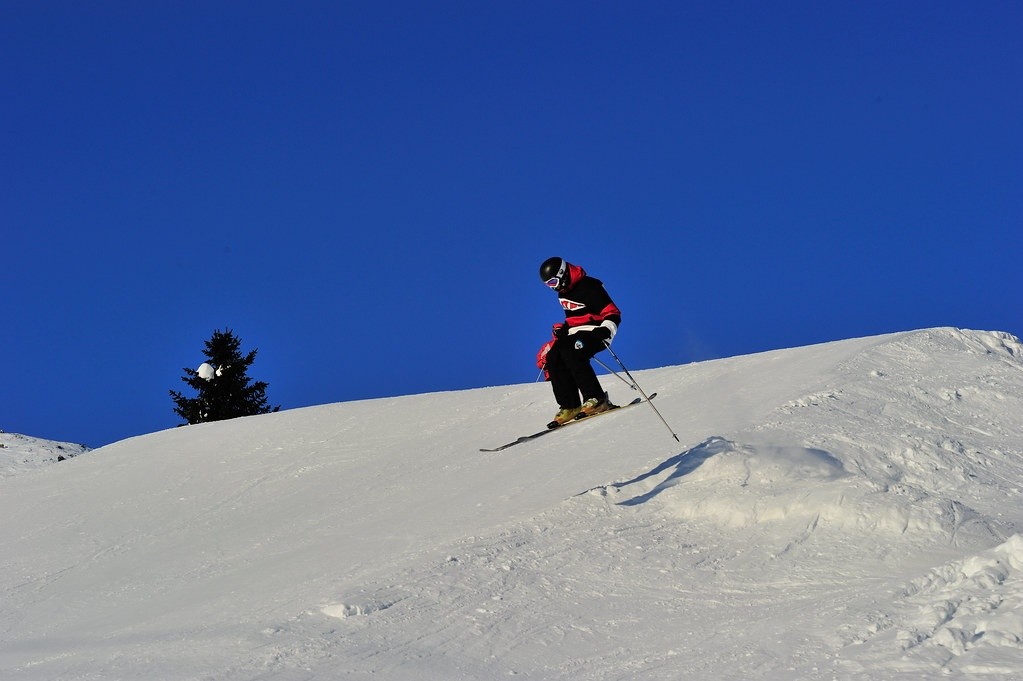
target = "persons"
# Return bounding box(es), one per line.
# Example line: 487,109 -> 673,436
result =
537,256 -> 622,429
536,323 -> 564,380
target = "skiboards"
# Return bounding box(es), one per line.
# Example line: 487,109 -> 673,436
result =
480,393 -> 657,452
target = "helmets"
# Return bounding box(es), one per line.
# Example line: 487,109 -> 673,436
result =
539,256 -> 571,290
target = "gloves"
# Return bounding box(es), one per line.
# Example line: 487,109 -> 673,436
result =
592,327 -> 611,342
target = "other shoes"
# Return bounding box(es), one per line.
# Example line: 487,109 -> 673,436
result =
555,406 -> 583,419
581,399 -> 599,411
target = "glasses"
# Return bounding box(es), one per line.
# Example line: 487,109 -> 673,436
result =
543,257 -> 565,288
